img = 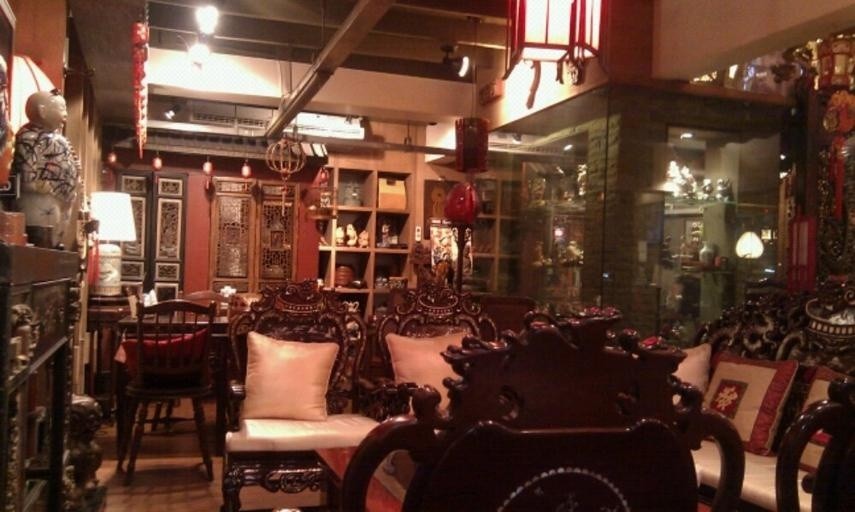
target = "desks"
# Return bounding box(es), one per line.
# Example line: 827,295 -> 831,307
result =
112,314 -> 230,474
313,447 -> 415,512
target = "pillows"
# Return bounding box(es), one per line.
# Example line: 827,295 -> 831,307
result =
124,329 -> 212,391
779,364 -> 855,475
670,341 -> 711,413
386,333 -> 473,415
236,331 -> 339,421
702,353 -> 798,455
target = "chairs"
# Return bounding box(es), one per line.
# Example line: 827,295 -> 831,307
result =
121,299 -> 224,486
136,291 -> 226,430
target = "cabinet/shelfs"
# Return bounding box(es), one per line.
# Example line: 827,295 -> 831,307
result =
318,152 -> 412,311
470,178 -> 516,300
0,245 -> 80,512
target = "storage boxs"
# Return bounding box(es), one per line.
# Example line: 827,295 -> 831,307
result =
378,178 -> 407,211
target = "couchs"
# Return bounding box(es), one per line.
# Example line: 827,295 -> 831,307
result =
359,290 -> 502,427
223,279 -> 399,512
340,307 -> 745,512
659,255 -> 855,512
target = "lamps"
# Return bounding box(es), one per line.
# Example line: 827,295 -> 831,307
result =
88,192 -> 136,296
164,104 -> 180,120
737,229 -> 765,286
440,39 -> 470,78
